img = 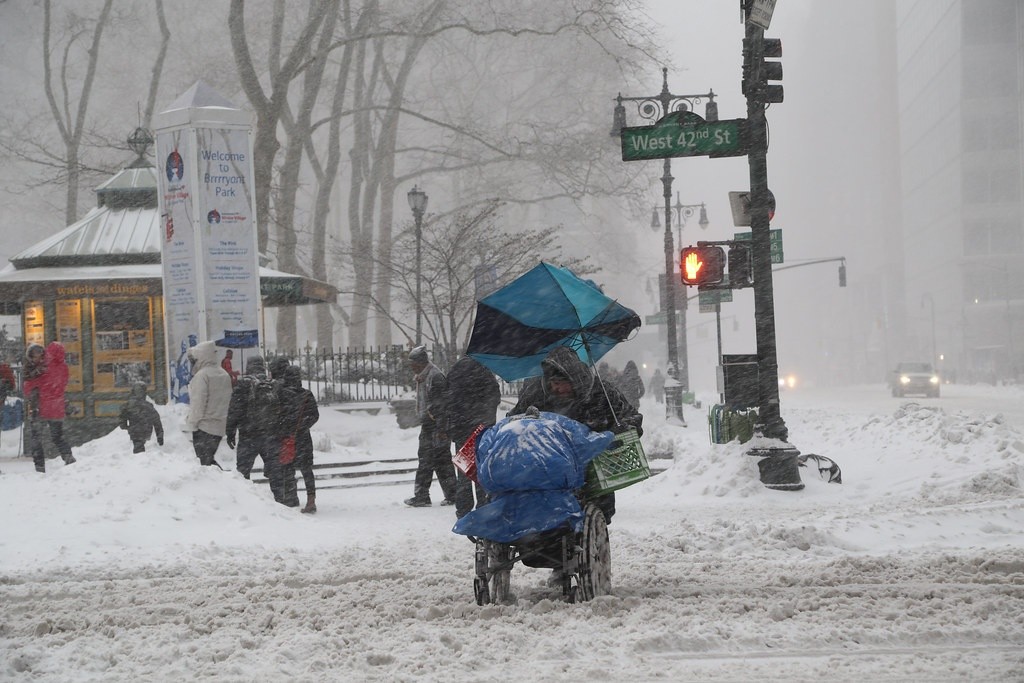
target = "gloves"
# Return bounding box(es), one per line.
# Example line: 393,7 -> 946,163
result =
157,437 -> 163,446
226,434 -> 236,449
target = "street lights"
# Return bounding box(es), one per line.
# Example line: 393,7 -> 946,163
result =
652,192 -> 710,402
407,185 -> 428,348
609,68 -> 720,429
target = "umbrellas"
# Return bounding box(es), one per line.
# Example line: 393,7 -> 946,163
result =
467,261 -> 642,425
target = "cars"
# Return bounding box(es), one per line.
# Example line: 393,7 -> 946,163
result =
891,359 -> 942,400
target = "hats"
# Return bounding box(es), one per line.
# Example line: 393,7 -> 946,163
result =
407,346 -> 428,361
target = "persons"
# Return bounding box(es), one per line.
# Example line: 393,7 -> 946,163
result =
649,367 -> 666,404
226,355 -> 285,502
21,341 -> 75,473
119,380 -> 164,453
505,346 -> 643,588
436,357 -> 502,520
599,361 -> 646,412
182,340 -> 233,471
221,349 -> 240,386
277,365 -> 320,514
404,349 -> 458,507
269,357 -> 289,389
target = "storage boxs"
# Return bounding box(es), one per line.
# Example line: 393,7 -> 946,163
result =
451,424 -> 486,485
581,429 -> 653,502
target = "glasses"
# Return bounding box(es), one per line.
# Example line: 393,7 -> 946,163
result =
189,358 -> 195,364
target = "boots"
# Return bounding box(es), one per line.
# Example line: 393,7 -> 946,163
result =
285,499 -> 299,506
301,495 -> 316,514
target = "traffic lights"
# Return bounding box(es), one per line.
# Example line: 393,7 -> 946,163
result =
739,38 -> 784,106
679,243 -> 725,288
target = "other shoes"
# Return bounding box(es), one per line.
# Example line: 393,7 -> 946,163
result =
64,453 -> 77,466
404,497 -> 432,507
34,463 -> 45,473
440,499 -> 454,506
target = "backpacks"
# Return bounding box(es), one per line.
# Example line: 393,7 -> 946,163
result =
246,373 -> 281,433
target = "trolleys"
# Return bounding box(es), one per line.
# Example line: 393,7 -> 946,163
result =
469,474 -> 613,605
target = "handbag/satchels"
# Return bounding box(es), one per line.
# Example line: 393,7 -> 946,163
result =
277,437 -> 296,466
